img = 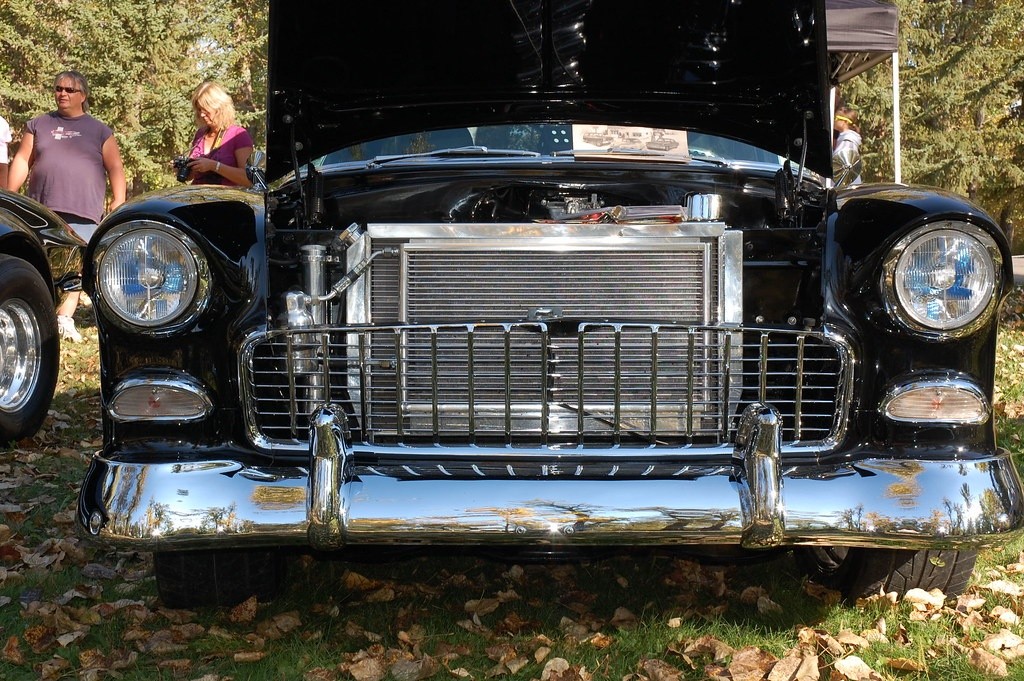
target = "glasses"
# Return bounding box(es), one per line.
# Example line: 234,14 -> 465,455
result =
55,86 -> 82,93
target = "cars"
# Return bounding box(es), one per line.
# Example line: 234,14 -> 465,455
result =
0,188 -> 94,444
76,1 -> 1019,606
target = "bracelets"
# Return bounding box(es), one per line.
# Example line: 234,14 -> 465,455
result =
215,162 -> 220,171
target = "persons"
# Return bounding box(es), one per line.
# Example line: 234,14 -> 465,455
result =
834,106 -> 862,184
170,82 -> 253,189
0,116 -> 12,187
7,71 -> 126,342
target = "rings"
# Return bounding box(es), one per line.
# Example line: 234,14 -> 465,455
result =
199,169 -> 200,171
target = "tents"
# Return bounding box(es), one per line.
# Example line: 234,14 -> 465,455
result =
825,0 -> 901,183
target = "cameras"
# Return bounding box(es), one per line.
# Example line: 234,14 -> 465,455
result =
174,157 -> 195,183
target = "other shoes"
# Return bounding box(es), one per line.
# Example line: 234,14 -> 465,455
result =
57,315 -> 82,343
